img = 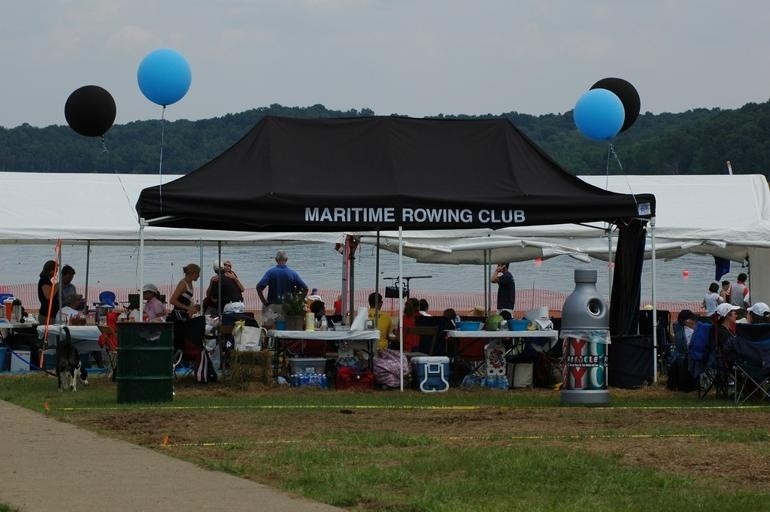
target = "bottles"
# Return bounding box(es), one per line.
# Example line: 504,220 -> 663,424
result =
321,314 -> 328,331
337,356 -> 365,372
62,310 -> 86,326
290,373 -> 328,391
11,298 -> 22,323
0,305 -> 5,318
345,312 -> 351,326
559,268 -> 612,405
143,311 -> 149,322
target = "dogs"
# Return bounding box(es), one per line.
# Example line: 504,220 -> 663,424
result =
56,327 -> 89,392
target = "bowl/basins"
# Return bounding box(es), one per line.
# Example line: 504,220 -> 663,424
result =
507,319 -> 529,330
454,320 -> 481,331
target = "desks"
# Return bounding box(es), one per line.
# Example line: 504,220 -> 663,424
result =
268,330 -> 380,384
37,324 -> 102,369
447,330 -> 559,390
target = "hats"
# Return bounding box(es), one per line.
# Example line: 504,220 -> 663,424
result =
213,259 -> 224,273
747,302 -> 770,318
678,310 -> 698,320
142,284 -> 158,294
715,303 -> 740,317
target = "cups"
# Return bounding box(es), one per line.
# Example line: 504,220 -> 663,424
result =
305,313 -> 314,330
364,320 -> 374,330
3,299 -> 14,321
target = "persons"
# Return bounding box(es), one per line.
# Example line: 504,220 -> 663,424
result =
490,262 -> 515,320
640,272 -> 770,397
257,250 -> 308,346
39,260 -> 250,349
310,285 -> 458,355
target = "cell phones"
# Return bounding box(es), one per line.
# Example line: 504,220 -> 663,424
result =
503,267 -> 505,269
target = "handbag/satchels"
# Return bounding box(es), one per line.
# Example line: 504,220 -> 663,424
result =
374,347 -> 413,388
241,326 -> 262,346
197,349 -> 218,383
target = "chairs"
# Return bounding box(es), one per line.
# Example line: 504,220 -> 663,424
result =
672,322 -> 770,407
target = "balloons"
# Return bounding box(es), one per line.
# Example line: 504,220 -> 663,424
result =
137,49 -> 192,107
588,78 -> 641,134
574,88 -> 626,141
64,85 -> 116,137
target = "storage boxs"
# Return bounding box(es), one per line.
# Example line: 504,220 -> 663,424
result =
411,356 -> 449,393
